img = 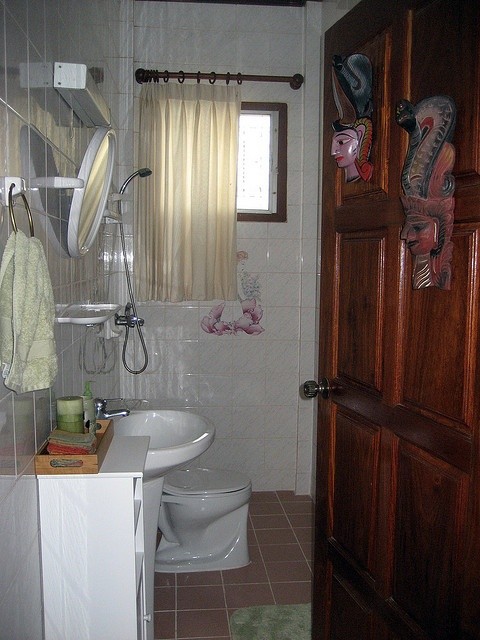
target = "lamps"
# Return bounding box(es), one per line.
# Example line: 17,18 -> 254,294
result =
54,61 -> 111,129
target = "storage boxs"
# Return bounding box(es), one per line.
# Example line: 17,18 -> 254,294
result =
34,419 -> 114,474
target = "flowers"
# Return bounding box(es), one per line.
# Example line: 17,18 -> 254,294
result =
200,249 -> 265,336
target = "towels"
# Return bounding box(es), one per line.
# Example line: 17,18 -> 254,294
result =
47,429 -> 95,447
0,229 -> 58,396
47,443 -> 89,455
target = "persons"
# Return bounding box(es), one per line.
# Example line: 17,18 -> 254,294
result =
329,116 -> 375,184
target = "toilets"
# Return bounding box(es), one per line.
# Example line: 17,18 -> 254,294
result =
153,467 -> 252,573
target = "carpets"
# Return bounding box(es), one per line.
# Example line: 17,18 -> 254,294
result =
228,601 -> 312,639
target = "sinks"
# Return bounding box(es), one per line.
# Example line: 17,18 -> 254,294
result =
120,410 -> 216,477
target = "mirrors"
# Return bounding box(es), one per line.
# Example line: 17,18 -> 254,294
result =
67,126 -> 118,259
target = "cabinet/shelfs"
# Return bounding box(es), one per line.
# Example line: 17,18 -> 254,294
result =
38,478 -> 151,640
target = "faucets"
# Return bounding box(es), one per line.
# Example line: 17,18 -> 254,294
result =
95,395 -> 130,420
